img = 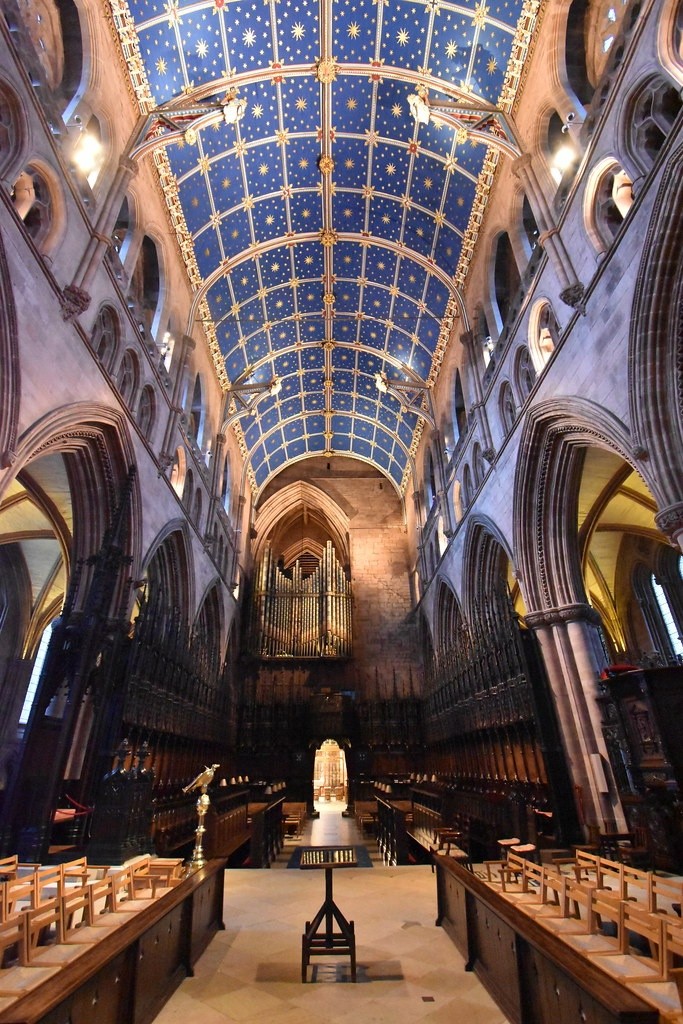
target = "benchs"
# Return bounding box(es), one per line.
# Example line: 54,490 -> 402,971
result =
354,800 -> 414,833
243,802 -> 307,838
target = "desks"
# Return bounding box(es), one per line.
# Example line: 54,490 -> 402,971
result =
599,832 -> 635,872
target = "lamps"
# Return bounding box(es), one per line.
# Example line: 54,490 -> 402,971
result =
265,781 -> 287,806
374,781 -> 391,804
484,336 -> 498,348
561,112 -> 583,134
202,450 -> 213,459
444,447 -> 454,455
410,773 -> 437,791
219,776 -> 250,795
65,115 -> 88,134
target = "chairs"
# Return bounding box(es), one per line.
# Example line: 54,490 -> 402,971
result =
0,854 -> 184,1013
430,827 -> 473,873
482,831 -> 683,1024
571,821 -> 651,873
